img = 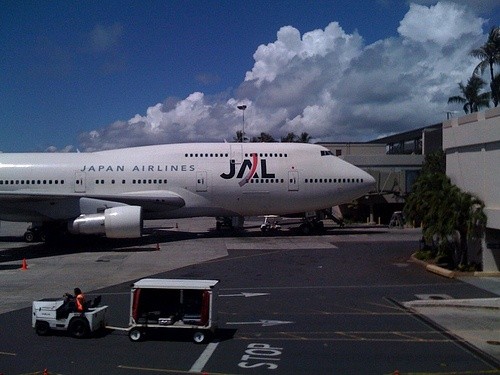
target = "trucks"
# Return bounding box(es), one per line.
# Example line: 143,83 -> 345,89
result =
31,293 -> 107,340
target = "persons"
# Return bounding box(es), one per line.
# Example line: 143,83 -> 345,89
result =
64,288 -> 87,318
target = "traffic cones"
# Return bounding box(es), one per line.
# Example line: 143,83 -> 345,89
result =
155,242 -> 160,250
20,259 -> 29,270
175,223 -> 178,228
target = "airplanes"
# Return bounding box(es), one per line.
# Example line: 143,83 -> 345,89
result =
0,143 -> 376,238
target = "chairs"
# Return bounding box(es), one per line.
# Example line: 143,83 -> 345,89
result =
82,300 -> 92,312
92,295 -> 101,308
